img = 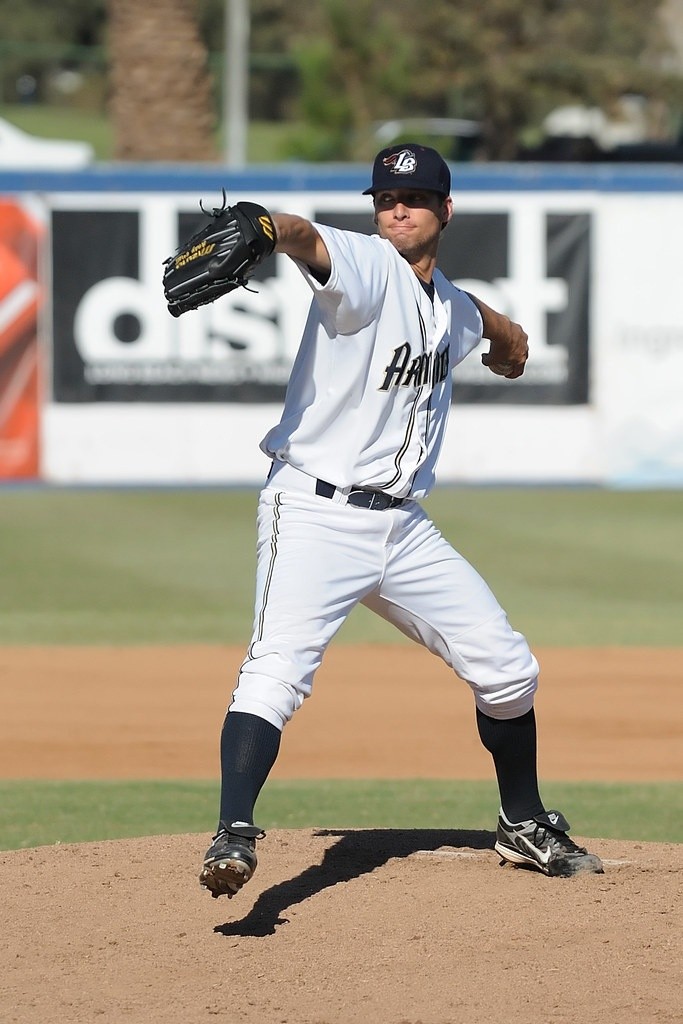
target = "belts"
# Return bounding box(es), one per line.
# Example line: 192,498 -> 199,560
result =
267,461 -> 406,509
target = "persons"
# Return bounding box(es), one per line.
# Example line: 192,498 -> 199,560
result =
456,73 -> 653,159
196,142 -> 607,900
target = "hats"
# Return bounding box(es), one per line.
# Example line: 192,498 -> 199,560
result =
362,143 -> 450,196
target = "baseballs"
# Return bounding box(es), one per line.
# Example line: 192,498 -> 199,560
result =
489,364 -> 513,376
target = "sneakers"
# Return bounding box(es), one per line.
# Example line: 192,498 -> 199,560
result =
495,807 -> 604,876
200,818 -> 266,899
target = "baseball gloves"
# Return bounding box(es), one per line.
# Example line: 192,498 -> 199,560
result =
160,185 -> 278,319
